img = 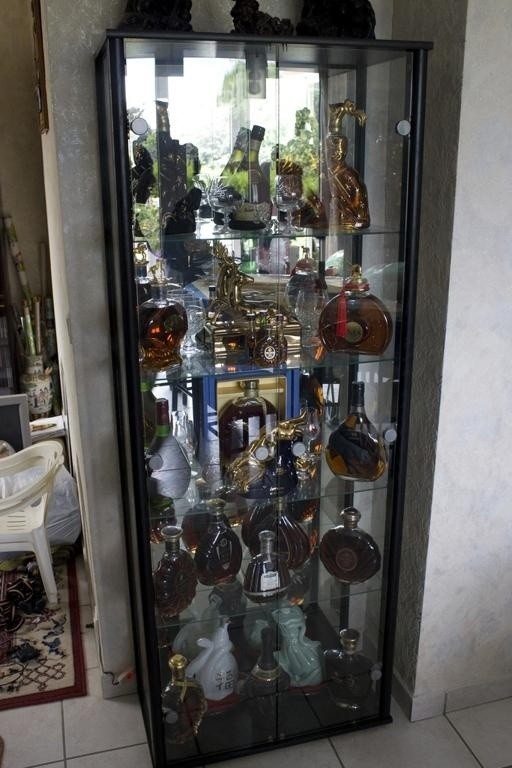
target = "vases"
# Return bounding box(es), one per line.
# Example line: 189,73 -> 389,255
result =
18,354 -> 55,414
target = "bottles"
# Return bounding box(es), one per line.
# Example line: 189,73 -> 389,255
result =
226,123 -> 273,230
252,326 -> 285,368
151,367 -> 388,742
285,248 -> 328,350
207,127 -> 253,216
139,380 -> 159,448
318,262 -> 392,356
208,286 -> 217,300
318,100 -> 371,230
137,282 -> 188,371
155,97 -> 197,234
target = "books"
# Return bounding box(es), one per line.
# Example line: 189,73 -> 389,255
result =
29,416 -> 67,444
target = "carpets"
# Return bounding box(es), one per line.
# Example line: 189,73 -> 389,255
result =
0,545 -> 88,711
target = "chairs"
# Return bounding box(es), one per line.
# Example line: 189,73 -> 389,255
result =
0,439 -> 65,604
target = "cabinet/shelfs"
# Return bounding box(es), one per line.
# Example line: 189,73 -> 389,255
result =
93,28 -> 434,768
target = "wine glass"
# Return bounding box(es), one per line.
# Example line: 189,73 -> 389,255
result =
207,172 -> 245,236
272,172 -> 306,235
167,289 -> 208,355
